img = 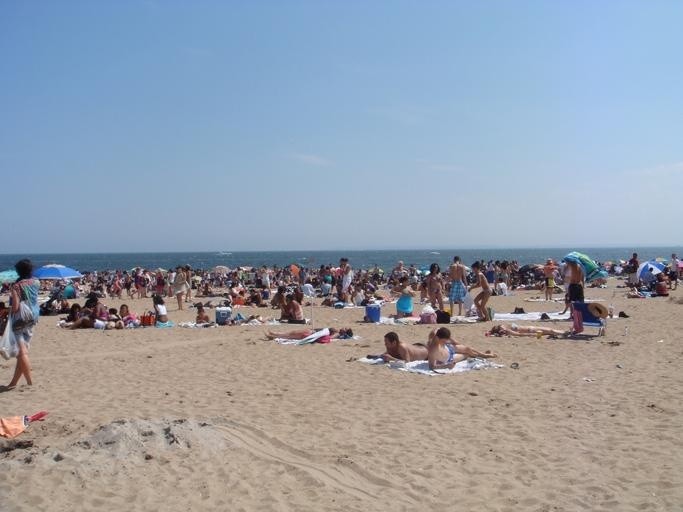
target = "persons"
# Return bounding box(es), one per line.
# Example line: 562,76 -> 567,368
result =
1,254 -> 683,390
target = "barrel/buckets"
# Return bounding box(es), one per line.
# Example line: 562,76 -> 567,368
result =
366,304 -> 381,321
216,307 -> 232,324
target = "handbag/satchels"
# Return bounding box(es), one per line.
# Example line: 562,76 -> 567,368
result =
12,301 -> 34,331
420,310 -> 450,324
140,309 -> 154,326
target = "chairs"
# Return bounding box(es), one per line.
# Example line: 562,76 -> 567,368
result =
568,301 -> 607,337
